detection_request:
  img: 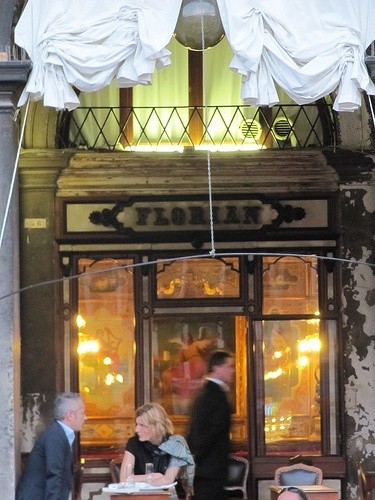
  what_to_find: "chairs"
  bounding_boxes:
[110,451,322,500]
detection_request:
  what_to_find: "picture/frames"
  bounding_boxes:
[151,318,240,418]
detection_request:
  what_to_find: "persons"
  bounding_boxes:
[277,487,307,500]
[119,403,195,500]
[187,351,235,500]
[17,392,86,500]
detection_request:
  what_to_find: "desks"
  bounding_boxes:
[268,485,338,500]
[105,481,178,500]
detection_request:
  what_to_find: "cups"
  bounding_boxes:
[126,464,134,488]
[145,463,154,484]
